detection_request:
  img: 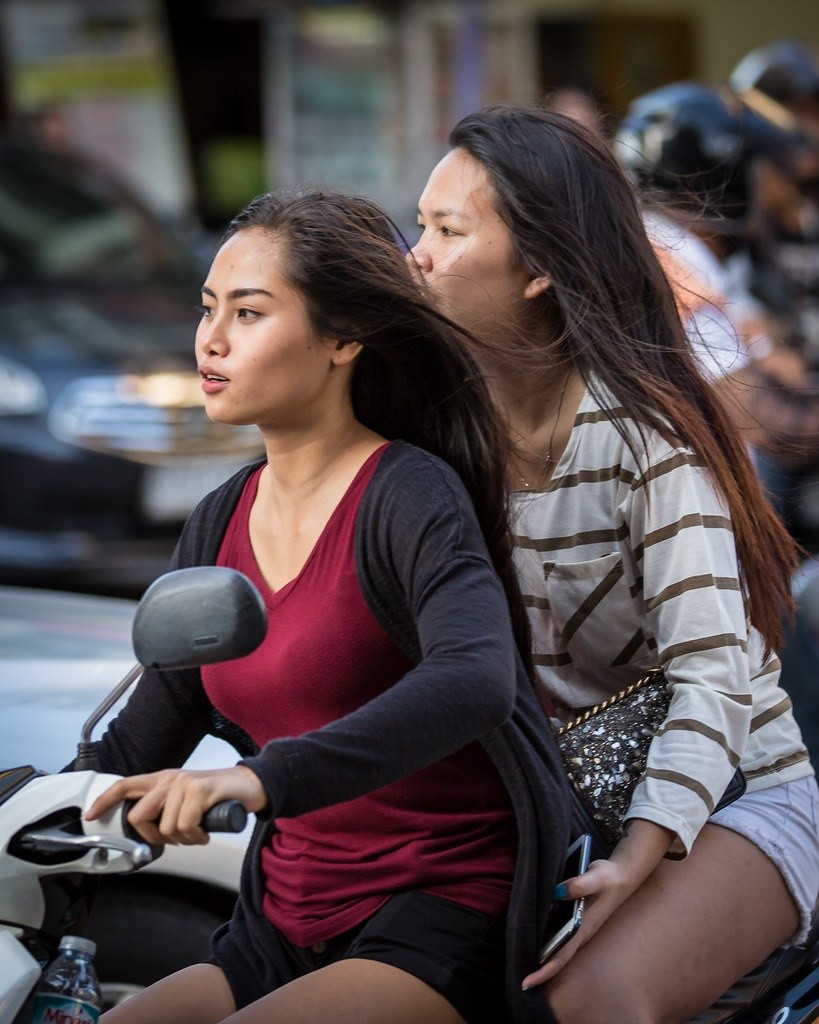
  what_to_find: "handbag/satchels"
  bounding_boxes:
[555,668,746,848]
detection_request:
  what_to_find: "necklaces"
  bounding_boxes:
[511,366,573,492]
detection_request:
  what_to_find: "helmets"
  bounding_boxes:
[729,40,818,141]
[616,86,760,226]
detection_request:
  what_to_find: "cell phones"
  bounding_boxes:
[539,834,593,966]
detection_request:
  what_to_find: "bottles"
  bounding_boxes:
[23,937,104,1024]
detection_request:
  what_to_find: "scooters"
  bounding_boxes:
[2,565,819,1024]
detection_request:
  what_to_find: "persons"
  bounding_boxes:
[404,105,819,1024]
[540,64,819,562]
[63,191,620,1024]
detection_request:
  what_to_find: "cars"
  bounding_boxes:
[0,125,268,594]
